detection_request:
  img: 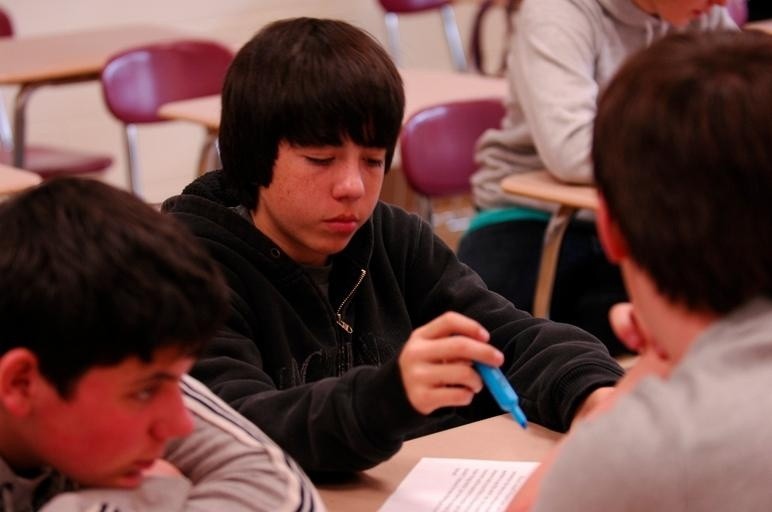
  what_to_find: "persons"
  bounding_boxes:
[504,27,772,512]
[0,177,327,512]
[459,0,743,360]
[160,18,625,487]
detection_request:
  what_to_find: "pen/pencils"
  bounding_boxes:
[450,332,528,430]
